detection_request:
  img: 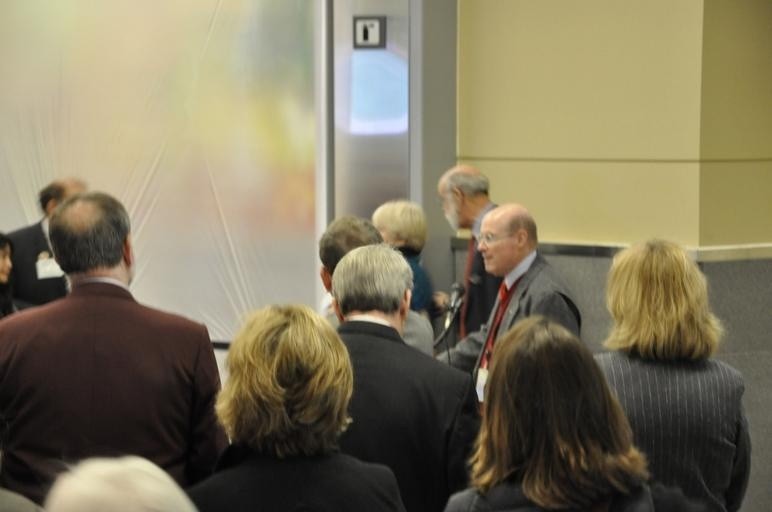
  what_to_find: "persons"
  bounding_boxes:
[0,191,230,507]
[370,199,434,328]
[318,215,434,356]
[437,165,505,350]
[5,177,88,305]
[435,204,582,415]
[445,313,656,512]
[42,454,199,512]
[331,243,480,511]
[186,301,406,512]
[593,238,752,512]
[0,232,14,317]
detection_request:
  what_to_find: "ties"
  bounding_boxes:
[457,235,475,341]
[480,275,524,369]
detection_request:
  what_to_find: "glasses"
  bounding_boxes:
[472,229,519,243]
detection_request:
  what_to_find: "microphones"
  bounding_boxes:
[446,282,465,311]
